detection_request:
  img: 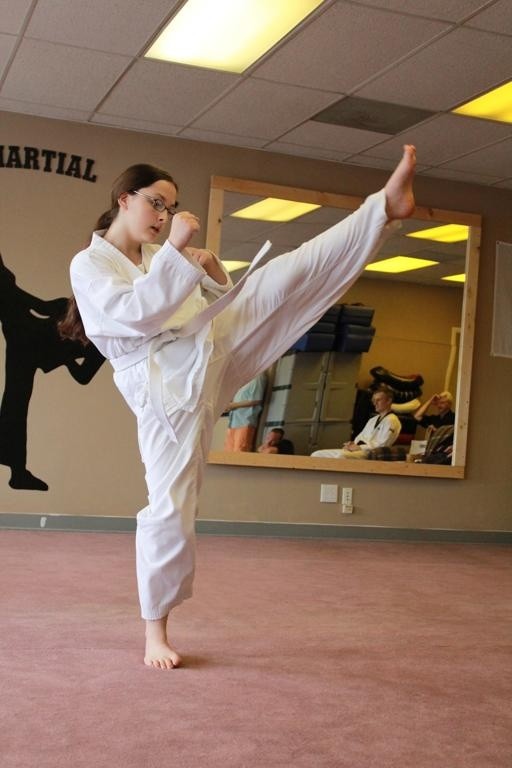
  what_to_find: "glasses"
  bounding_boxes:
[133,190,177,214]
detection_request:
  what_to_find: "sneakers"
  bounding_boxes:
[9,470,49,490]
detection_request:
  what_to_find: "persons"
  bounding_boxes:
[70,144,421,668]
[415,391,455,447]
[311,386,403,462]
[224,372,267,452]
[0,254,107,491]
[257,428,294,455]
[422,433,452,465]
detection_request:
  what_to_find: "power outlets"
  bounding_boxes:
[342,488,354,514]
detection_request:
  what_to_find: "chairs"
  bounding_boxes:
[367,433,416,461]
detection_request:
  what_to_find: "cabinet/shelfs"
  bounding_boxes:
[261,351,363,456]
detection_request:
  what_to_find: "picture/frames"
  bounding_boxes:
[489,240,512,359]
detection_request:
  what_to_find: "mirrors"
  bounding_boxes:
[202,173,484,481]
